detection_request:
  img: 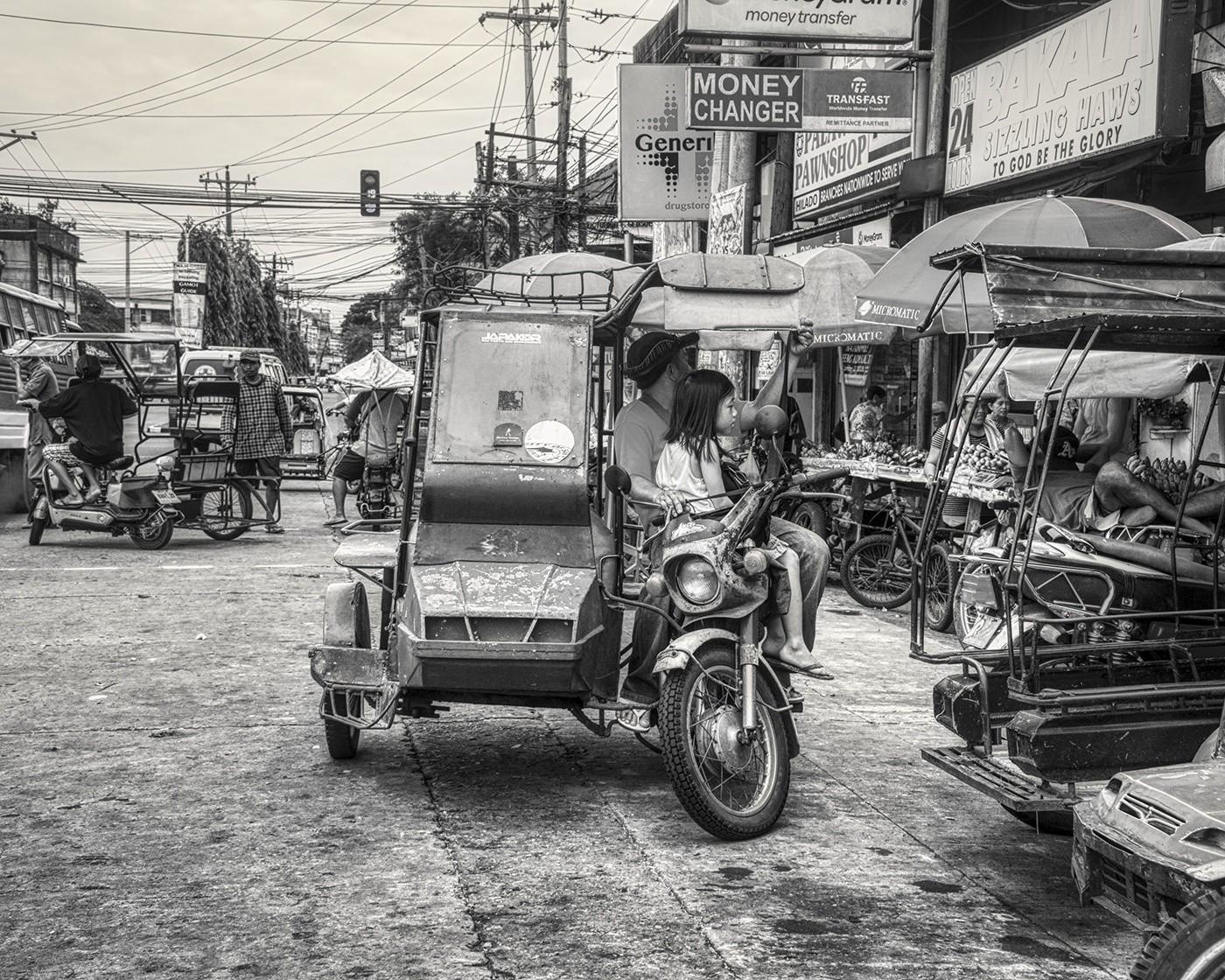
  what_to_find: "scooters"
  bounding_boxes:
[326,406,404,532]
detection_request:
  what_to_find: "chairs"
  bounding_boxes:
[1008,517,1225,662]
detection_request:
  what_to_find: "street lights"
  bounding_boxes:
[101,183,275,263]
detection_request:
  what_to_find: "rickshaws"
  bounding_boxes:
[913,242,1225,831]
[15,330,282,550]
[303,249,857,843]
[1067,707,1224,980]
[172,373,330,481]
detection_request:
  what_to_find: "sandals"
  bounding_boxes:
[1119,506,1156,527]
[1196,537,1225,561]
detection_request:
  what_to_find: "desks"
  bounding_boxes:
[798,455,943,540]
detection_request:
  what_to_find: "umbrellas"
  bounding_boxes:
[624,189,1225,401]
[454,250,650,321]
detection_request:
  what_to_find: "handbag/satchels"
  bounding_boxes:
[831,418,851,443]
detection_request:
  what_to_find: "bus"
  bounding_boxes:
[0,281,84,513]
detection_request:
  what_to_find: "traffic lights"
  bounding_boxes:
[360,169,381,216]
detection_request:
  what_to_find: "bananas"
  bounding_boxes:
[1126,455,1210,495]
[799,432,929,468]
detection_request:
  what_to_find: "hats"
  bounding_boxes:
[239,349,261,361]
[76,355,101,374]
[1,339,41,355]
[1037,425,1080,471]
[623,332,701,381]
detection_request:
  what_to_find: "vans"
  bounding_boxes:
[168,348,294,438]
[122,327,183,399]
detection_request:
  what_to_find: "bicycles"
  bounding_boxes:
[840,483,958,632]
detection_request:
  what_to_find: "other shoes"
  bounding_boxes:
[240,522,252,532]
[265,525,284,533]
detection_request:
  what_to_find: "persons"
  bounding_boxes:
[219,348,293,534]
[2,339,60,530]
[293,376,318,429]
[831,385,1133,561]
[323,386,408,525]
[613,332,834,733]
[23,353,140,508]
[1002,424,1224,567]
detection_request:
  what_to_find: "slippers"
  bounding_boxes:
[615,697,651,733]
[781,663,833,679]
[323,518,347,528]
[783,685,805,703]
[84,495,106,506]
[764,656,787,667]
[50,498,83,507]
